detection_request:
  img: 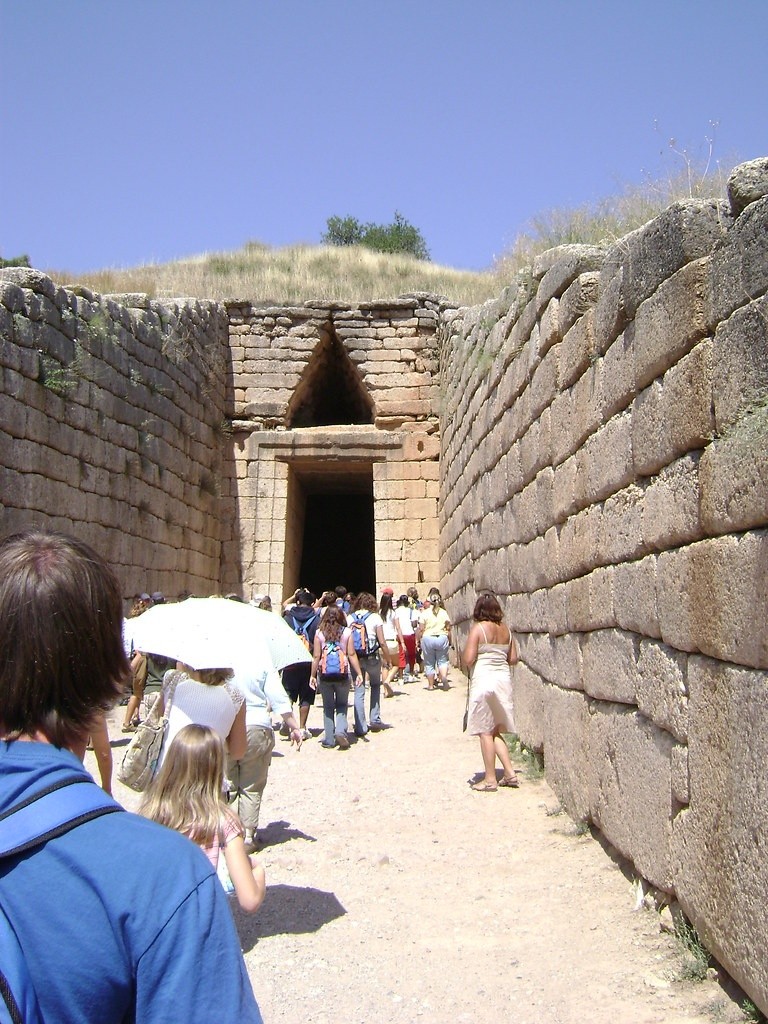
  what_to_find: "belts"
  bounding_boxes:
[386,638,398,641]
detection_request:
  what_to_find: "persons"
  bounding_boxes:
[135,725,266,913]
[151,660,304,873]
[462,592,519,791]
[278,585,456,751]
[0,535,259,1024]
[122,592,272,732]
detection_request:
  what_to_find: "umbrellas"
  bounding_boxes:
[126,597,315,678]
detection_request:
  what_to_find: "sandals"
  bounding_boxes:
[498,776,520,788]
[472,779,497,791]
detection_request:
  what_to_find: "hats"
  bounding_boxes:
[140,593,151,601]
[225,592,241,599]
[250,594,265,607]
[427,595,440,603]
[380,586,394,594]
[153,592,164,600]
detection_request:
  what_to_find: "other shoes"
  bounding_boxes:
[370,723,390,732]
[336,732,350,749]
[121,723,138,733]
[442,677,450,691]
[244,836,265,854]
[132,720,144,727]
[383,682,394,698]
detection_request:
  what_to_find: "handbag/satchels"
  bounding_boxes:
[463,711,468,732]
[117,669,185,793]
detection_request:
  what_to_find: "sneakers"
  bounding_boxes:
[409,675,420,682]
[279,720,290,736]
[398,679,404,686]
[299,726,312,740]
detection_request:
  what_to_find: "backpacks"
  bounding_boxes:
[284,615,317,673]
[350,611,380,656]
[320,626,348,680]
